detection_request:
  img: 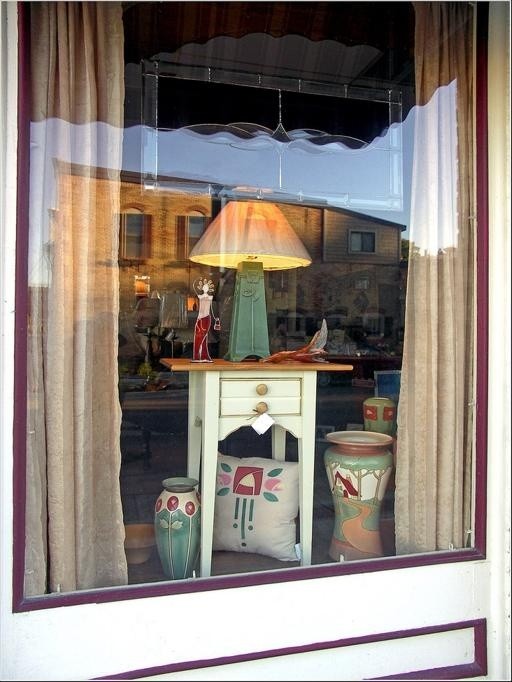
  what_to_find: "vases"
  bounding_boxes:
[321,430,393,562]
[363,397,396,433]
[152,477,200,578]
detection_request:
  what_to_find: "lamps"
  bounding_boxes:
[186,200,314,361]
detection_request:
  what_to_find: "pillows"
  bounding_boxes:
[212,448,300,564]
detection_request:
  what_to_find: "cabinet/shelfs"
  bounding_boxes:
[158,357,353,577]
